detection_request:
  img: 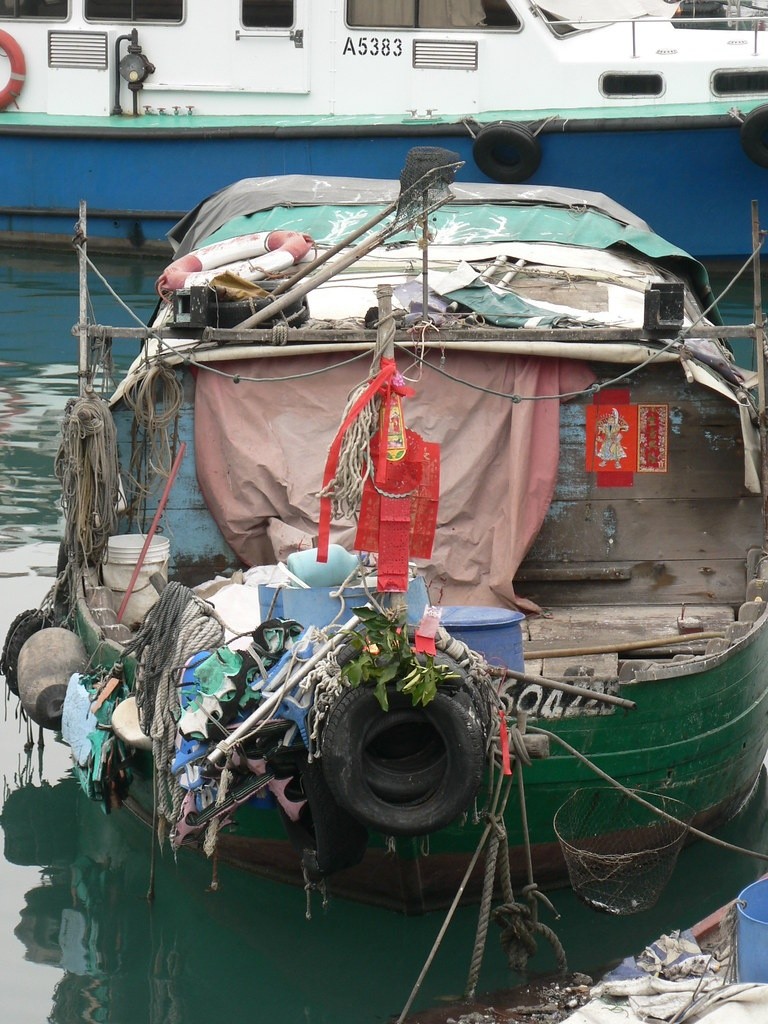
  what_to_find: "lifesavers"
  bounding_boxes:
[156,226,313,300]
[263,644,489,878]
[0,30,26,113]
[470,119,539,182]
[741,107,768,168]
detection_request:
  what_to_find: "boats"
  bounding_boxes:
[0,0,768,264]
[68,174,765,916]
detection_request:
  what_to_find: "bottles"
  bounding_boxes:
[286,543,371,587]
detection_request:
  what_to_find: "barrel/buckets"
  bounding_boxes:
[281,574,432,626]
[736,877,768,983]
[257,583,282,626]
[427,604,526,678]
[100,534,171,628]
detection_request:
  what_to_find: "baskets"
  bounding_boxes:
[553,787,697,917]
